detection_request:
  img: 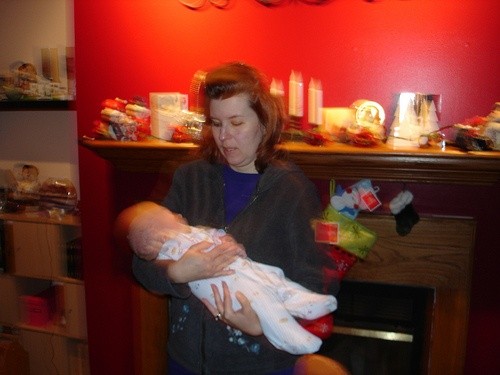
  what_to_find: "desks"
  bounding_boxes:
[77,137,500,375]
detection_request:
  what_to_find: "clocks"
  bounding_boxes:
[350,99,386,127]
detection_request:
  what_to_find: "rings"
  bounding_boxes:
[216,313,221,320]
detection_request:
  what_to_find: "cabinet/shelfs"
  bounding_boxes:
[0,99,86,343]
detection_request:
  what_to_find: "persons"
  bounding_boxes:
[107,62,322,375]
[113,198,337,354]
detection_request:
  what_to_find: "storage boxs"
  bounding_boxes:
[150,91,187,141]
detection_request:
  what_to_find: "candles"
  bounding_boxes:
[271,70,322,128]
